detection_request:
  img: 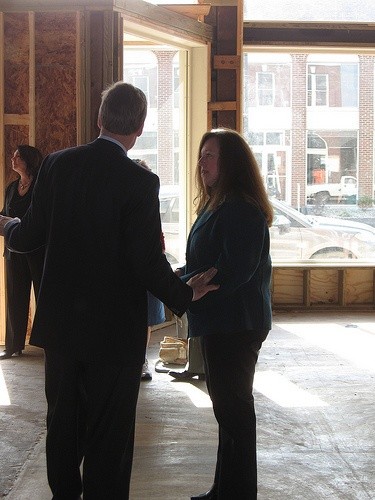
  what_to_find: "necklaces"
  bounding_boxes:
[19,181,29,190]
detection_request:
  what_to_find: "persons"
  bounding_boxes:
[0,143,43,359]
[0,81,219,500]
[130,127,273,500]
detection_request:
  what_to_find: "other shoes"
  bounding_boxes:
[140,370,152,380]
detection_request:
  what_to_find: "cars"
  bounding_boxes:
[158,184,375,268]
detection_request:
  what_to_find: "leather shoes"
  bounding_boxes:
[169,370,205,380]
[191,483,220,500]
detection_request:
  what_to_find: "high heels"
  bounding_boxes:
[0,347,22,359]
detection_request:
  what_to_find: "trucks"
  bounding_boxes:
[305,176,358,206]
